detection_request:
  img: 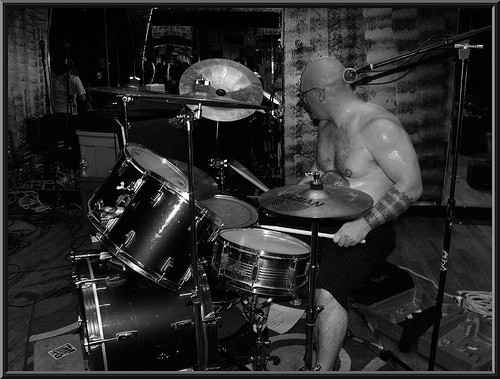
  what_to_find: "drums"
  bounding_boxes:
[194,192,258,228]
[208,227,313,301]
[79,141,191,234]
[70,250,221,372]
[98,170,225,294]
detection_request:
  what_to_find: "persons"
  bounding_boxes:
[299,58,423,371]
[50,58,86,115]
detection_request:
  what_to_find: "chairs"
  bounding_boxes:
[344,265,415,359]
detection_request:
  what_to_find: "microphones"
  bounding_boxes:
[342,67,357,83]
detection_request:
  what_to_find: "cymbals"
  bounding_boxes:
[82,84,157,97]
[167,158,219,201]
[138,94,272,110]
[258,182,375,220]
[177,58,266,123]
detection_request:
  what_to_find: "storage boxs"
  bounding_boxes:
[76,175,105,223]
[75,128,118,176]
[368,286,492,371]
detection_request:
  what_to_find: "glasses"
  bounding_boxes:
[298,88,325,100]
